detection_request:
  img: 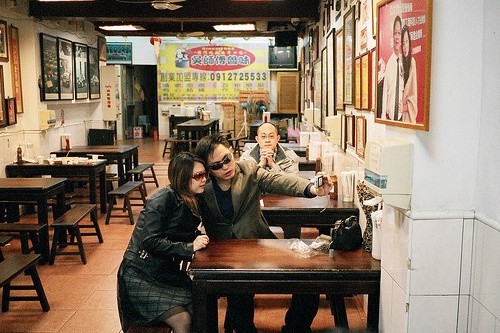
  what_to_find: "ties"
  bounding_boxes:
[394,58,399,120]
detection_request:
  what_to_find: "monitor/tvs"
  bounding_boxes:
[268,45,296,68]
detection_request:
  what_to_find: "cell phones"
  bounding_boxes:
[314,175,323,187]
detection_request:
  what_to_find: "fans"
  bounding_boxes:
[117,0,187,10]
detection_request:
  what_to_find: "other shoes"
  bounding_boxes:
[281,324,311,333]
[225,324,258,333]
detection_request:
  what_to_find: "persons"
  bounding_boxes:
[239,122,302,240]
[193,133,332,333]
[381,16,418,124]
[117,152,210,333]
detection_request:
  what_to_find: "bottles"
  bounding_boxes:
[17,147,23,164]
[264,114,267,122]
[306,145,309,160]
[197,106,204,120]
[65,136,70,150]
[329,175,337,200]
[315,157,321,176]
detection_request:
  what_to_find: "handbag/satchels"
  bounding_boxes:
[334,215,362,250]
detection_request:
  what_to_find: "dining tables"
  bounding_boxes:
[0,144,140,261]
[189,142,381,332]
[176,117,220,148]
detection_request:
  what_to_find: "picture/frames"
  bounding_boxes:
[0,20,100,127]
[299,0,433,157]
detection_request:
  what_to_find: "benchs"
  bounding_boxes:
[160,132,247,157]
[0,162,159,311]
[125,323,171,333]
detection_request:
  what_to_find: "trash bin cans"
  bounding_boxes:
[89,128,116,161]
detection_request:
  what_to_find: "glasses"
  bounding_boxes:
[191,170,207,182]
[208,151,232,171]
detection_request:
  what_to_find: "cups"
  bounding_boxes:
[51,154,56,159]
[92,155,98,161]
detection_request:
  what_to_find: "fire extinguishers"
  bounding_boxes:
[154,129,157,140]
